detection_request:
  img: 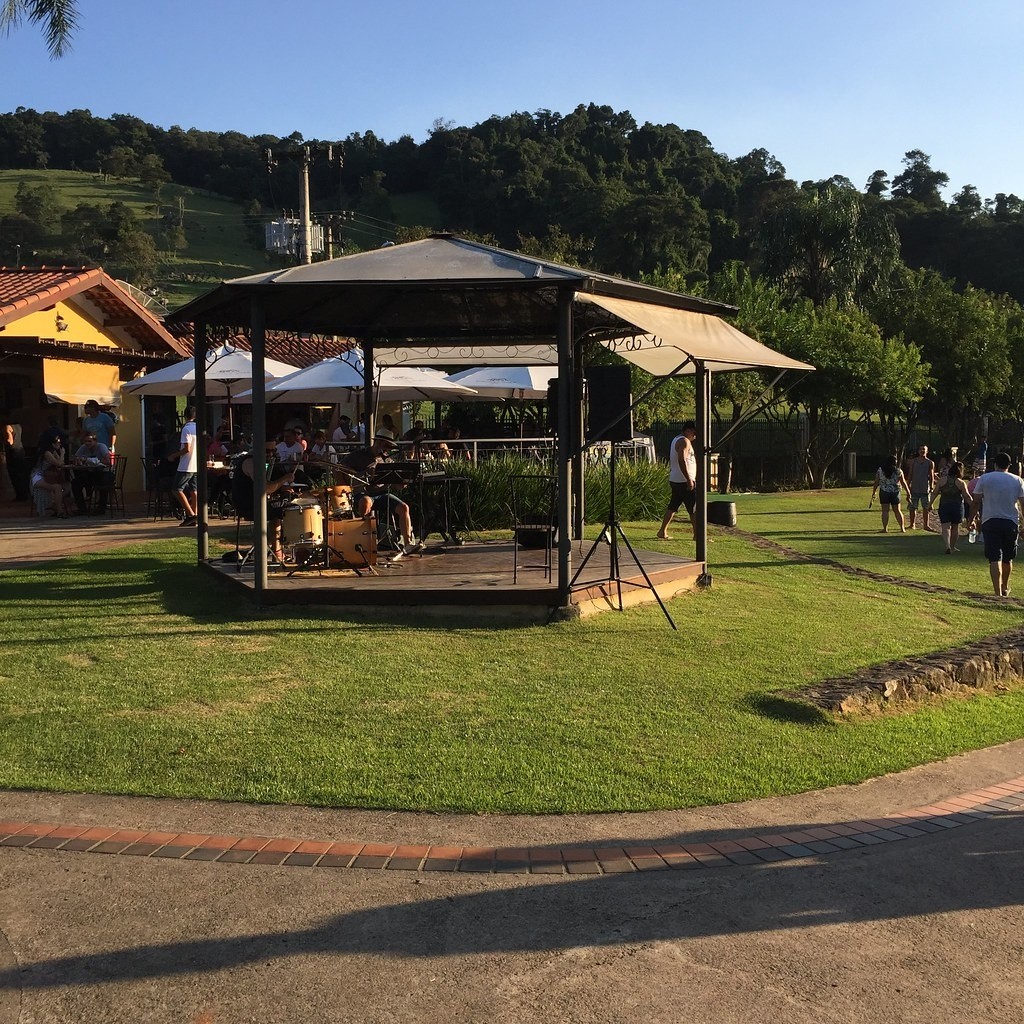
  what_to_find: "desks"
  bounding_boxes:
[408,478,470,547]
[58,465,110,516]
[207,468,228,478]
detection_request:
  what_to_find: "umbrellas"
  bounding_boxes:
[120,338,302,441]
[445,364,559,462]
[234,344,479,451]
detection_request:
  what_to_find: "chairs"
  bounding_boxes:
[88,453,127,518]
[509,475,559,584]
[140,457,173,523]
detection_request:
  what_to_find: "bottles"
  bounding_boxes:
[968,529,976,543]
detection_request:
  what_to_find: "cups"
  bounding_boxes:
[287,452,308,462]
[209,454,214,462]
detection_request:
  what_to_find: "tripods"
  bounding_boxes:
[369,434,440,558]
[238,460,362,576]
[546,443,677,632]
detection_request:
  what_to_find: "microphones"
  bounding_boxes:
[355,544,363,554]
[378,448,386,463]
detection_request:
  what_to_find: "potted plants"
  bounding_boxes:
[515,496,558,549]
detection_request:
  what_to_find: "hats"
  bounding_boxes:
[370,428,397,446]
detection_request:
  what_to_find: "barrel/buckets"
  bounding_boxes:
[707,501,737,526]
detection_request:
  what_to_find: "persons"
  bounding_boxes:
[656,421,700,539]
[937,440,1024,529]
[927,461,973,553]
[32,400,473,556]
[872,453,912,533]
[965,452,1024,597]
[905,445,936,531]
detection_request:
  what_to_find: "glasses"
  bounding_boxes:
[295,431,302,436]
[266,447,277,454]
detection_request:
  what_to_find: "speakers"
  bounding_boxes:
[587,364,633,443]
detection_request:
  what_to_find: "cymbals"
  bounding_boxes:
[277,458,304,464]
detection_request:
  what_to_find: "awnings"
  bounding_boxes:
[371,343,565,439]
[571,283,817,458]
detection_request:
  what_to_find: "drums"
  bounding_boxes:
[326,515,377,568]
[282,495,323,548]
[311,485,352,517]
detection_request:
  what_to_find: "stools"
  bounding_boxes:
[237,514,286,573]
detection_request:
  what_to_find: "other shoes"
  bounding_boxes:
[272,550,294,563]
[187,521,196,526]
[179,515,197,527]
[403,542,423,556]
[1002,588,1012,596]
